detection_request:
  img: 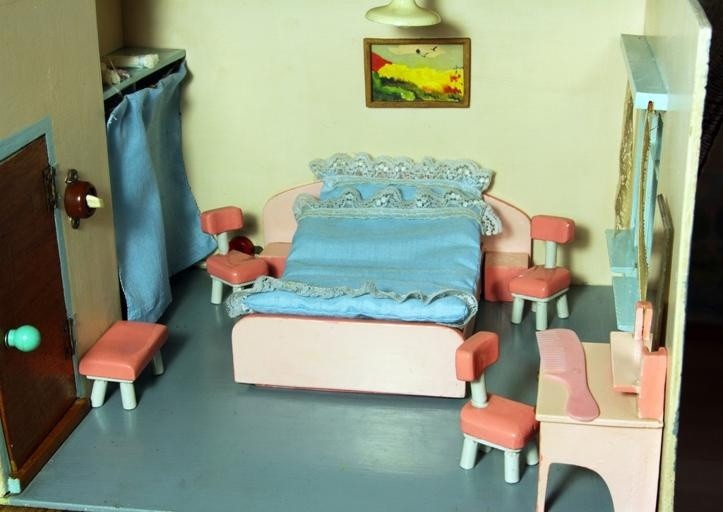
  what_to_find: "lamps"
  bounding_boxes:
[363,0,440,28]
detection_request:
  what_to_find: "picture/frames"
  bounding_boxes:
[363,37,471,107]
